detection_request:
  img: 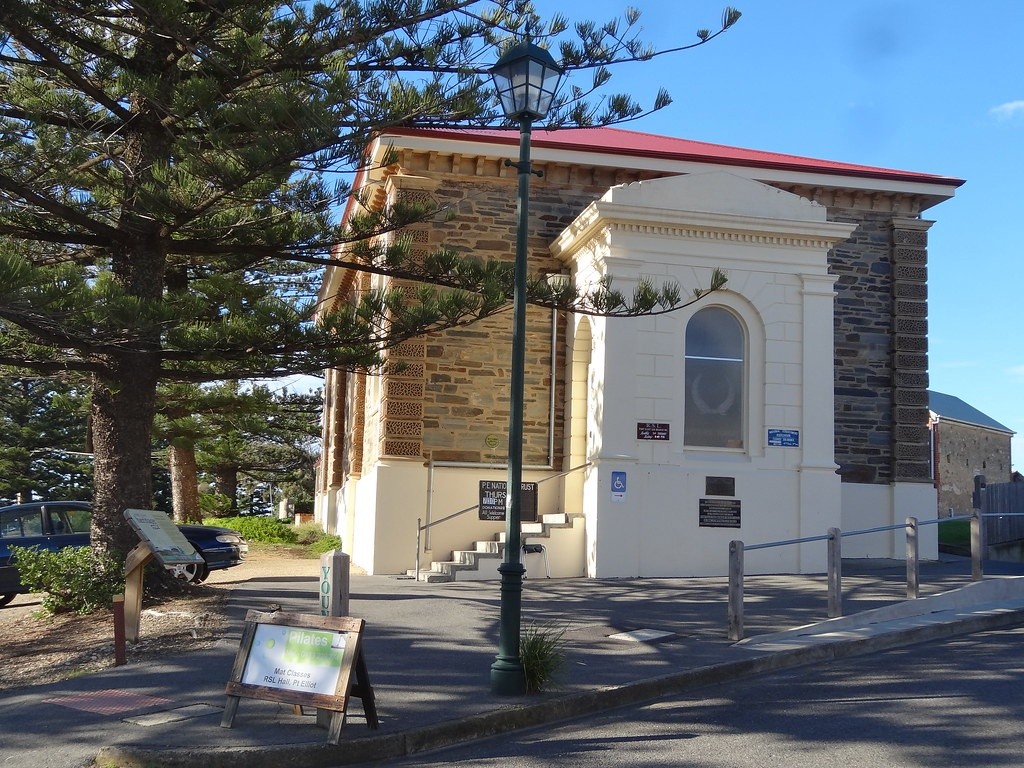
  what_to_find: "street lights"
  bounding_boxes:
[269,482,273,517]
[488,19,566,693]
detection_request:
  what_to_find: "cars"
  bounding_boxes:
[0,501,248,609]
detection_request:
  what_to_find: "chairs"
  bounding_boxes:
[56,521,71,533]
